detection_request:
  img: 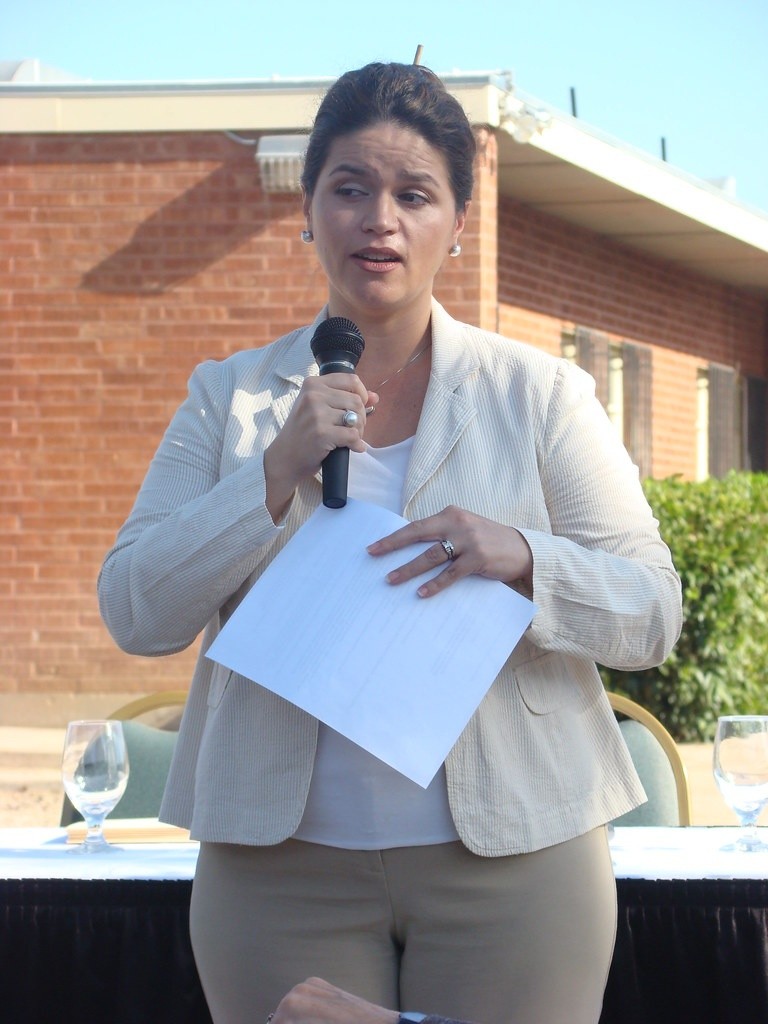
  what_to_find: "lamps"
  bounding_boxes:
[255,133,308,194]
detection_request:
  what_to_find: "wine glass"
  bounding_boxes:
[62,720,130,856]
[711,715,768,849]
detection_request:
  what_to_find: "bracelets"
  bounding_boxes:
[398,1012,474,1024]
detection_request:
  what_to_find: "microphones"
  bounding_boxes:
[309,316,365,511]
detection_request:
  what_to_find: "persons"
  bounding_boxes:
[97,62,683,1024]
[270,976,476,1024]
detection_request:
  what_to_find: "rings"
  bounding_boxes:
[440,539,455,560]
[342,408,358,428]
[265,1013,274,1024]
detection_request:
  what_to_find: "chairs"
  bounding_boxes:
[61,689,188,826]
[605,690,689,825]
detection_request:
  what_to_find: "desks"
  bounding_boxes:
[0,816,768,1024]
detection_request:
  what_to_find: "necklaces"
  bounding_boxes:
[365,343,432,415]
